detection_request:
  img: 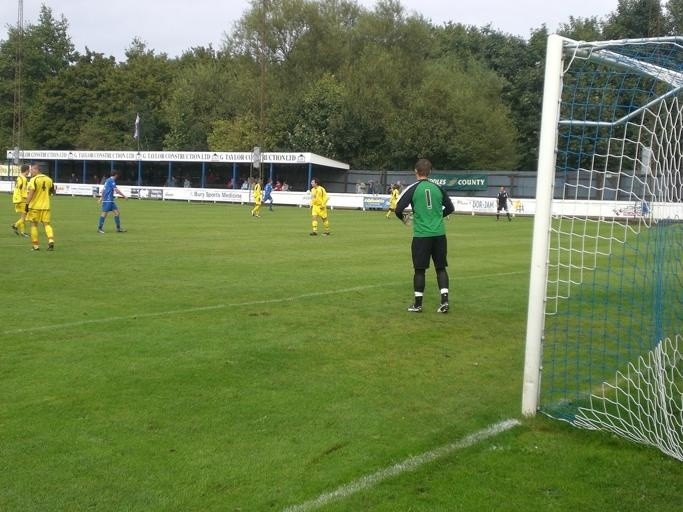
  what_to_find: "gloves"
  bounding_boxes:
[24,204,28,212]
[402,212,415,227]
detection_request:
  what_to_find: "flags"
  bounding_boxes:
[133,113,140,138]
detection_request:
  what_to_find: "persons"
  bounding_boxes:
[250,179,261,218]
[494,186,513,221]
[10,165,30,238]
[308,177,329,236]
[69,171,295,191]
[95,169,128,233]
[385,185,400,219]
[24,163,56,252]
[354,178,402,211]
[260,179,274,211]
[393,158,456,313]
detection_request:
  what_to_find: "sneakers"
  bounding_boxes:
[20,232,30,238]
[96,228,105,234]
[114,227,127,233]
[321,232,330,236]
[45,240,55,252]
[436,303,449,313]
[28,245,40,252]
[11,223,19,236]
[406,304,423,313]
[309,231,318,236]
